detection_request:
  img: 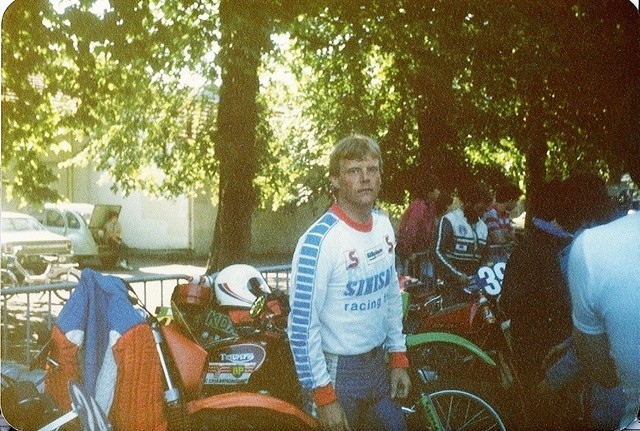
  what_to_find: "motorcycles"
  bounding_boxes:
[154,266,303,406]
[398,273,530,429]
[1,266,322,431]
[55,279,312,422]
[0,349,121,360]
[397,290,514,430]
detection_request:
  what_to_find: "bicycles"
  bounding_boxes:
[0,245,81,301]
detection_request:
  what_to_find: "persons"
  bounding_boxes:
[286,135,412,430]
[500,177,574,431]
[553,132,640,431]
[434,171,461,242]
[499,189,523,235]
[398,173,442,279]
[432,183,490,283]
[104,211,129,269]
[532,175,565,220]
[517,171,625,431]
[482,182,515,266]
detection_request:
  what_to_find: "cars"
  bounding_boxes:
[1,211,73,274]
[27,203,122,270]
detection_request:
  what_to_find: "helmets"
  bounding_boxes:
[214,262,271,308]
